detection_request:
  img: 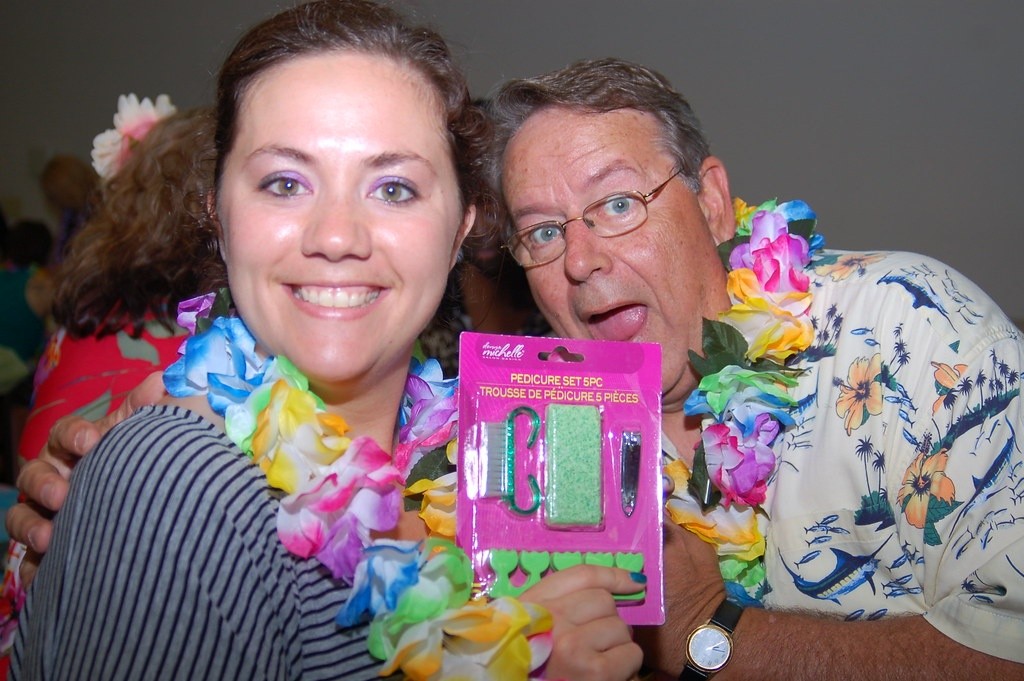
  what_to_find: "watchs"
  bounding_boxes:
[678,595,747,681]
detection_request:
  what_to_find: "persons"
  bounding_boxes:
[5,56,1024,681]
[0,0,645,681]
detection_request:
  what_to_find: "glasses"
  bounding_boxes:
[502,168,687,267]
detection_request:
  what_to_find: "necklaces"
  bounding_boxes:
[163,289,555,681]
[658,195,824,608]
